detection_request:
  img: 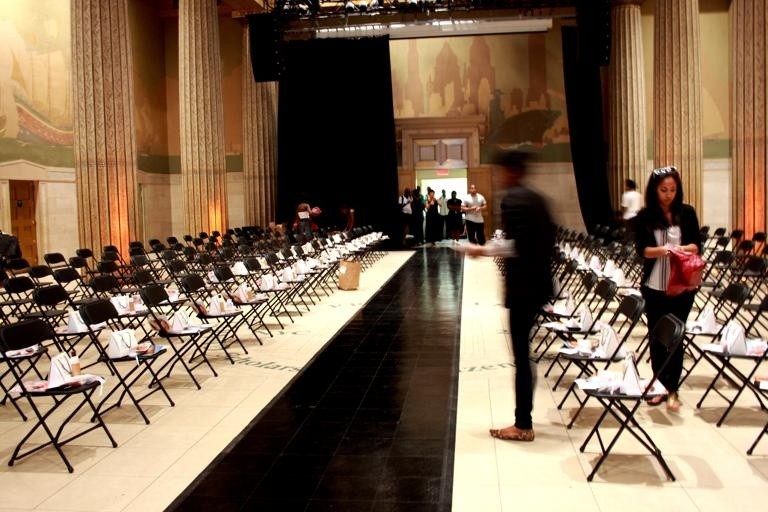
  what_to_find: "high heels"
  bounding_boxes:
[647,389,669,405]
[667,393,679,411]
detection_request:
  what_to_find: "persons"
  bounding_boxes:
[463,148,556,442]
[424,186,431,204]
[412,189,425,247]
[417,185,425,210]
[398,186,413,242]
[619,177,643,231]
[438,189,450,240]
[424,189,444,242]
[461,183,488,246]
[636,164,702,413]
[291,203,313,246]
[446,190,464,239]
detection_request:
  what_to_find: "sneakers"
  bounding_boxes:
[490,425,534,441]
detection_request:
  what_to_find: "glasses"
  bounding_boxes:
[653,166,676,180]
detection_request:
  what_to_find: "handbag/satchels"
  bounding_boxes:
[306,232,383,269]
[260,274,274,291]
[599,321,624,358]
[668,249,703,296]
[721,319,747,355]
[50,351,71,385]
[338,260,360,290]
[109,329,138,358]
[620,351,643,397]
[283,266,293,280]
[581,304,595,331]
[110,296,130,315]
[172,310,190,332]
[68,311,88,332]
[556,242,625,288]
[296,259,309,274]
[298,211,309,219]
[210,293,225,315]
[565,292,578,315]
[238,284,248,303]
